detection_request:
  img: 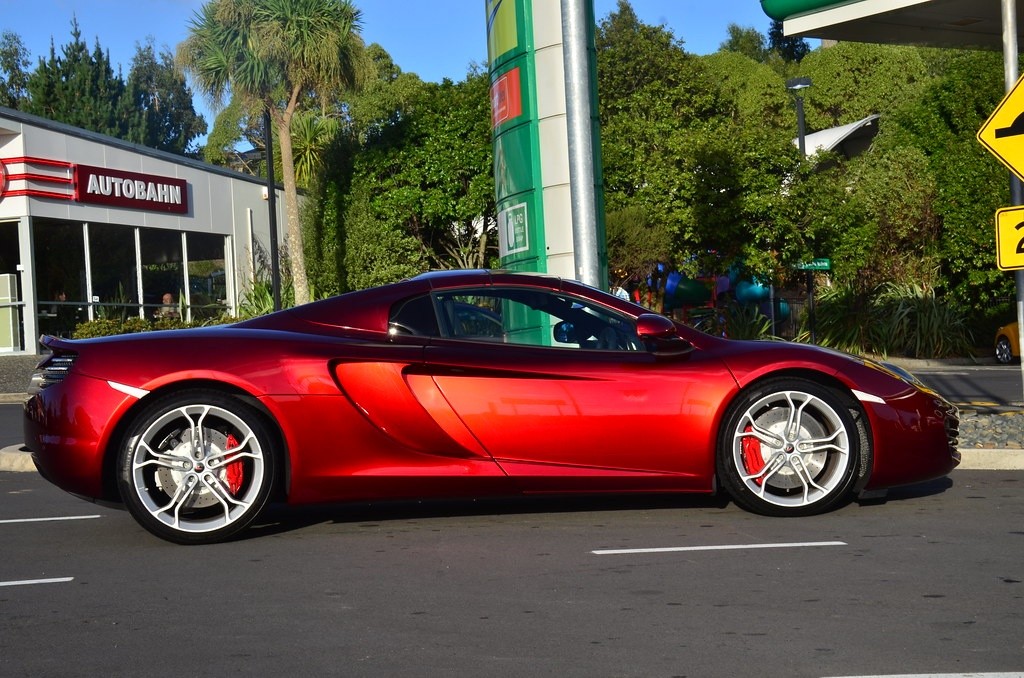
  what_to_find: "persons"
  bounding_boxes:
[51,290,67,337]
[161,293,180,314]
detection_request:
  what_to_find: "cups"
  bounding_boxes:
[42,310,47,318]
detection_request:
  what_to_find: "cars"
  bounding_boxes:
[993,320,1020,363]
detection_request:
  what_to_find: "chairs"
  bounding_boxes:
[441,296,466,337]
[45,300,76,339]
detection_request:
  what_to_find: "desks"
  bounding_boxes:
[37,313,79,338]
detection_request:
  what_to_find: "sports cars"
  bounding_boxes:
[19,270,963,546]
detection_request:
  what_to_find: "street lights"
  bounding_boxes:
[786,75,817,345]
